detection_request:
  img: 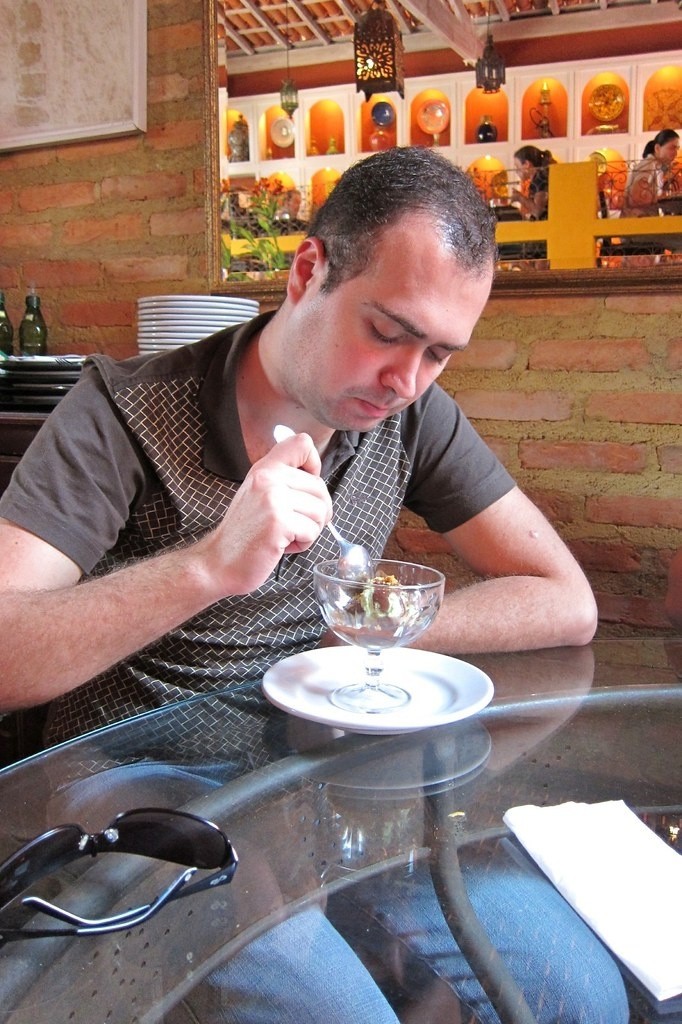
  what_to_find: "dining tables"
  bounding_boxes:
[0,635,682,1024]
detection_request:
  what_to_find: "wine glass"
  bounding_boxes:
[311,558,445,715]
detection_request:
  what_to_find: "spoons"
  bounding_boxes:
[273,423,374,589]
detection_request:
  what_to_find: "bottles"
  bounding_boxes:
[0,291,13,359]
[17,280,50,357]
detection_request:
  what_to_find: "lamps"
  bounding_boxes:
[353,0,404,98]
[280,0,298,118]
[475,0,506,95]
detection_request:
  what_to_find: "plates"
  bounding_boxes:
[418,99,448,134]
[589,82,626,121]
[370,102,394,127]
[261,645,496,735]
[270,118,294,148]
[136,295,261,365]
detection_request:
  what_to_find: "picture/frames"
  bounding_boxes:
[0,0,149,152]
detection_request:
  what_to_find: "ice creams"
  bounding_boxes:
[342,574,420,619]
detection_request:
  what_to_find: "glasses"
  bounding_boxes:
[0,807,239,938]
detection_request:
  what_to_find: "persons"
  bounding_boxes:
[511,145,557,260]
[0,147,632,1023]
[620,128,679,249]
